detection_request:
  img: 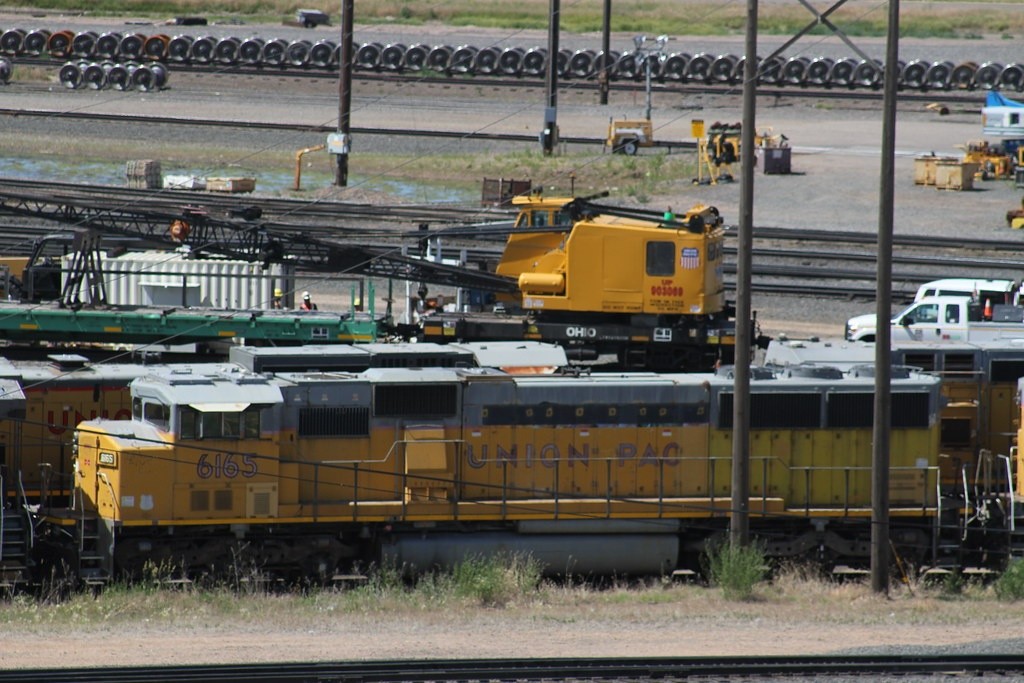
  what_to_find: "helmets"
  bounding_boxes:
[275,289,283,297]
[354,298,360,306]
[302,291,313,300]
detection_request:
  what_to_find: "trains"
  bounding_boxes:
[1,341,1024,580]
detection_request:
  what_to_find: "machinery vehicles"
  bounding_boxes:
[1,175,774,375]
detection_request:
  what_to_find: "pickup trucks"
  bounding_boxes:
[845,279,1024,364]
